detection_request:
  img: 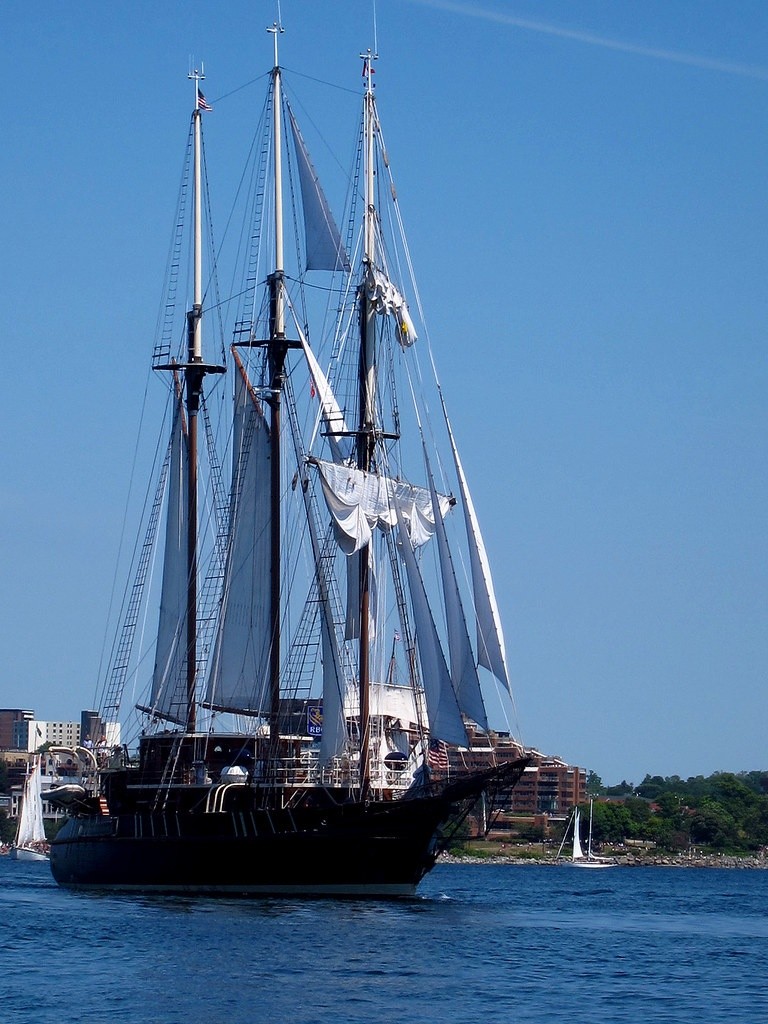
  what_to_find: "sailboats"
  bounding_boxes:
[11,753,48,861]
[38,0,534,899]
[562,806,619,869]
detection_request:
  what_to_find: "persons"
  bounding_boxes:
[429,739,448,772]
[83,734,108,764]
